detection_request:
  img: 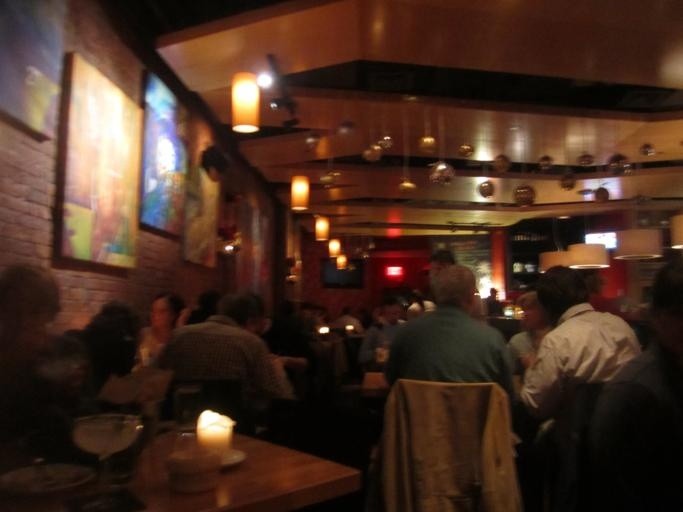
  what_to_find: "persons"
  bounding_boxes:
[0,249,683,511]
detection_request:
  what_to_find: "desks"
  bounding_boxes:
[0,425,361,512]
[484,314,528,342]
[359,372,522,403]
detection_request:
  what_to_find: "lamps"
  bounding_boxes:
[336,255,348,270]
[314,215,331,241]
[328,238,342,258]
[230,37,262,133]
[291,160,311,211]
